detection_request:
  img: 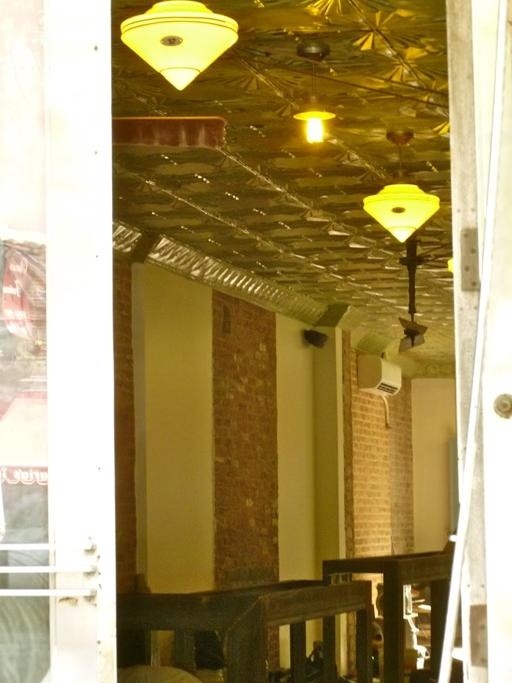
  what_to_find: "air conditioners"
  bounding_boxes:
[356,355,404,397]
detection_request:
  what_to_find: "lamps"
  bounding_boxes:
[293,45,337,144]
[121,2,240,91]
[362,130,439,239]
